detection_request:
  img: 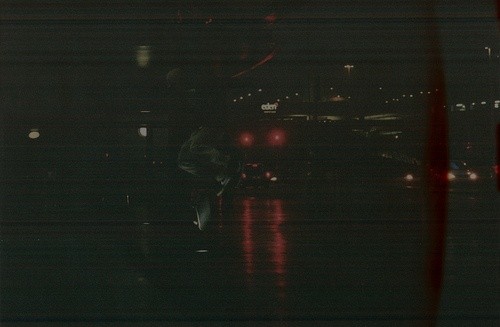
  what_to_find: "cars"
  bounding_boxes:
[399,163,497,188]
[239,164,273,188]
